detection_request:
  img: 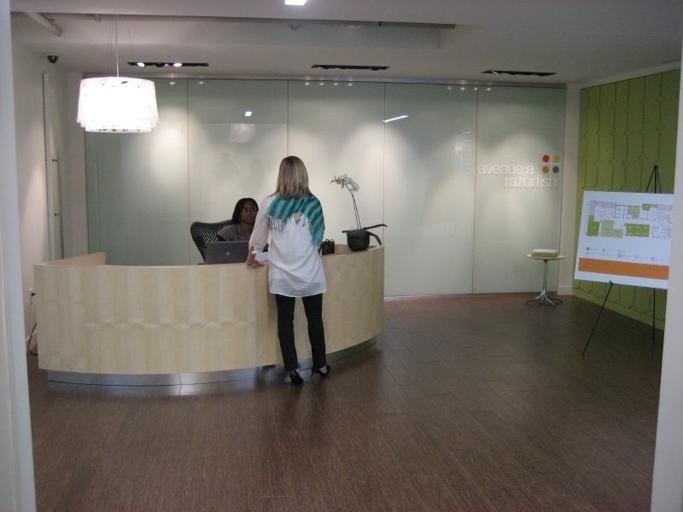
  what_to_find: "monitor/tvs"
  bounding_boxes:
[204,241,249,264]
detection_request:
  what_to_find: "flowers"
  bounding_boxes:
[329,173,388,245]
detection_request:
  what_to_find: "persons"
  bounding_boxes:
[246,155,331,387]
[215,195,259,242]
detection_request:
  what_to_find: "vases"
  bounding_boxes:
[347,231,370,251]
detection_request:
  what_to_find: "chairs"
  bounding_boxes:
[191,220,237,264]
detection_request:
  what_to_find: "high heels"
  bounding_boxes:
[310,365,331,378]
[289,371,303,385]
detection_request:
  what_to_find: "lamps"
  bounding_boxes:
[76,20,159,133]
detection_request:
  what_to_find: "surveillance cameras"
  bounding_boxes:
[47,55,59,65]
